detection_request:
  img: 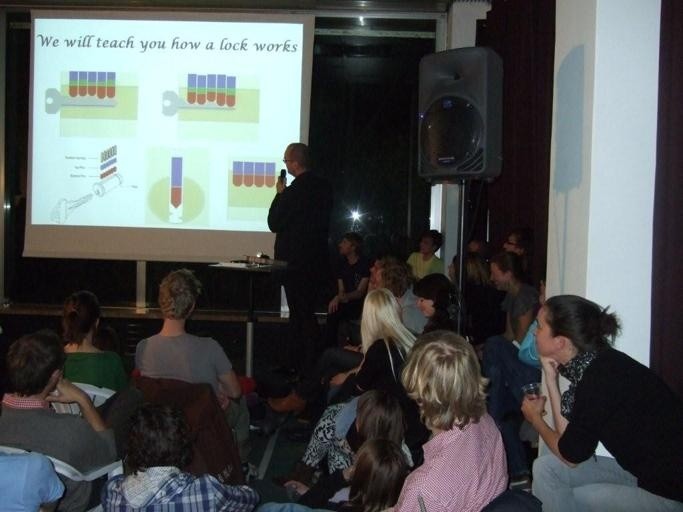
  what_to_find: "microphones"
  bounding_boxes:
[280,169,286,178]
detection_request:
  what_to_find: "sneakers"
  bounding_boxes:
[508,477,532,493]
[288,425,313,445]
[217,461,259,486]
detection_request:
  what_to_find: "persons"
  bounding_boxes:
[99,394,262,511]
[57,288,129,417]
[260,226,546,512]
[133,268,254,474]
[522,294,682,511]
[266,141,322,323]
[0,449,65,511]
[0,327,121,511]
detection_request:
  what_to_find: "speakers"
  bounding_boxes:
[417,46,503,186]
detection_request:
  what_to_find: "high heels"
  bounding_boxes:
[266,392,307,417]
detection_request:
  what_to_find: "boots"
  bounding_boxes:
[272,462,315,490]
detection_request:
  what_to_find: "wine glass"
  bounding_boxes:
[520,382,546,417]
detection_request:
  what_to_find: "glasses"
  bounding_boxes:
[505,238,521,248]
